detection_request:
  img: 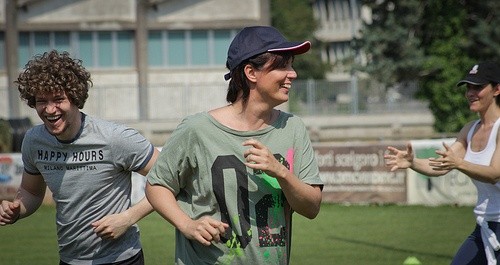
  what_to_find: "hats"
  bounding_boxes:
[456,60,500,87]
[224,25,312,80]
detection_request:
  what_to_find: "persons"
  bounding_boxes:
[0,48,162,265]
[382,64,500,265]
[145,25,325,265]
